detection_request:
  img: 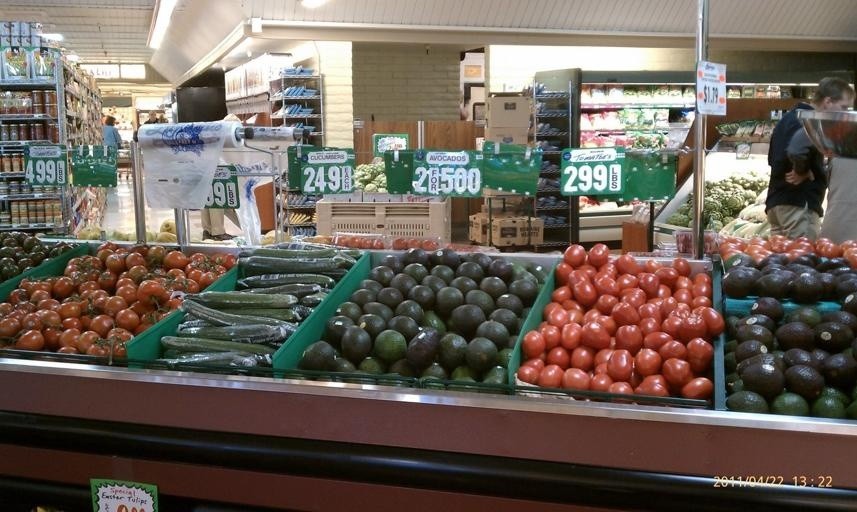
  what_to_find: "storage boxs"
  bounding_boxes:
[485,95,531,126]
[469,213,489,244]
[484,128,529,146]
[483,198,528,215]
[487,217,544,248]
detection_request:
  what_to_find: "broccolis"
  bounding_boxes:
[664,171,769,232]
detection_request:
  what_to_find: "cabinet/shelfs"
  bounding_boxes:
[2,236,857,508]
[2,43,73,240]
[579,83,696,250]
[528,79,572,252]
[268,73,325,147]
[274,152,321,241]
[62,59,108,237]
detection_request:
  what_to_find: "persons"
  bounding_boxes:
[818,154,857,239]
[765,77,857,239]
[103,116,122,146]
[136,110,166,125]
[202,209,234,241]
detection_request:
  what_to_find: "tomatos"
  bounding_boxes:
[0,240,235,356]
[522,244,727,402]
[722,236,853,268]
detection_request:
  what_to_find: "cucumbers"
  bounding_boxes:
[144,240,364,371]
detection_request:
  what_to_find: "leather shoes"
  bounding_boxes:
[209,232,236,243]
[202,229,212,240]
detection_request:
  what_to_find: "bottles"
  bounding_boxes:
[0,123,59,143]
[0,152,25,172]
[0,20,43,48]
[33,91,43,104]
[0,91,33,114]
[45,104,57,115]
[45,90,57,104]
[33,104,44,114]
[0,181,62,195]
[0,199,63,224]
